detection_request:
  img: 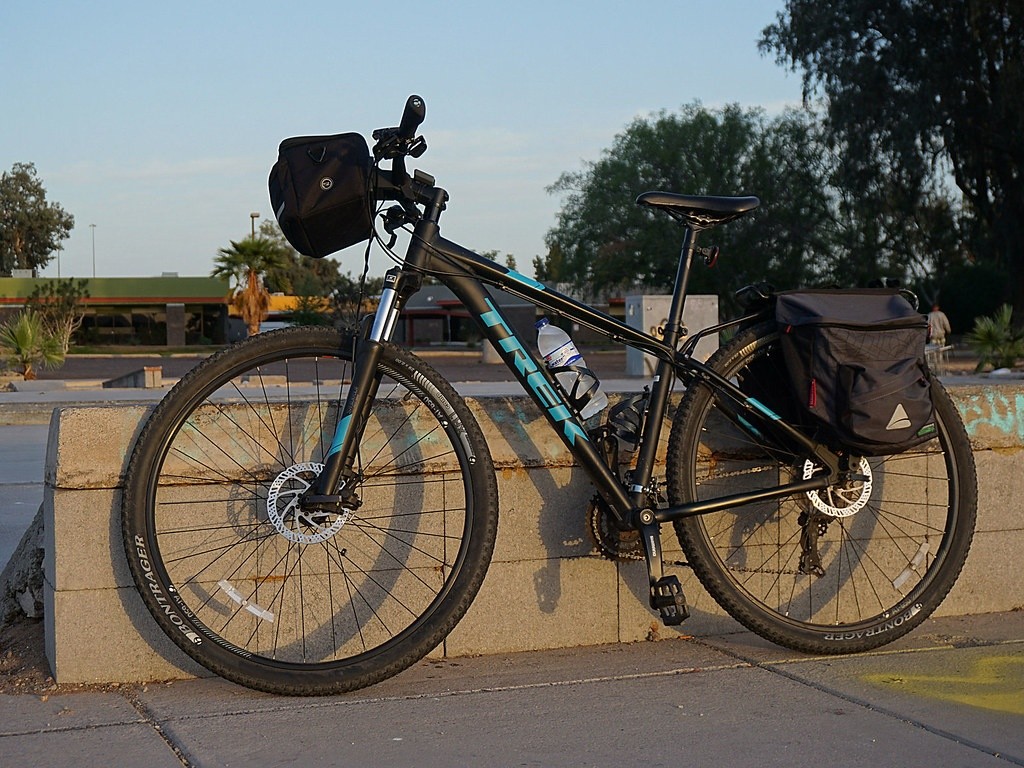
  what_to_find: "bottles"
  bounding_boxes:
[535,316,610,420]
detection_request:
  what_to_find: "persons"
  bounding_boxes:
[926,305,951,362]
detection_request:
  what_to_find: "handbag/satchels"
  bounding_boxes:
[267,132,375,259]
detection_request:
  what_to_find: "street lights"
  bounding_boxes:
[251,212,259,240]
[89,224,96,278]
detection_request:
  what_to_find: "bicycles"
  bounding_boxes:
[120,94,980,701]
